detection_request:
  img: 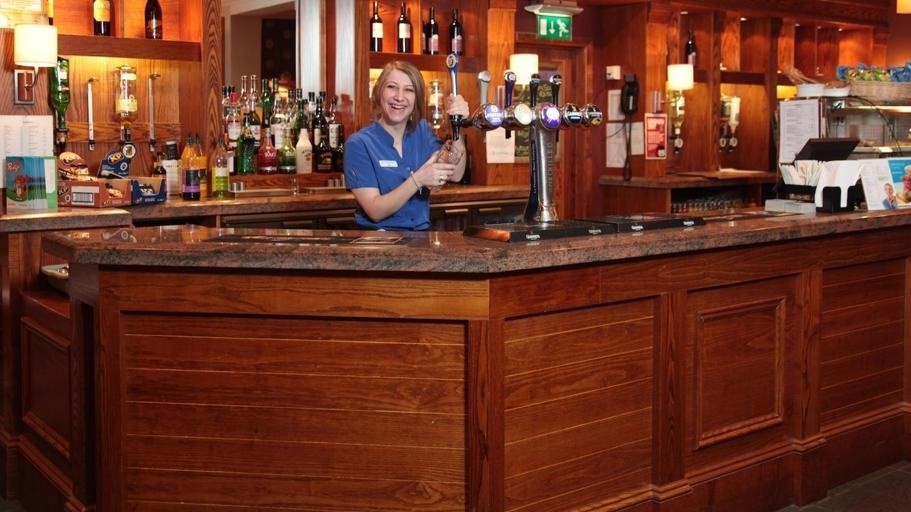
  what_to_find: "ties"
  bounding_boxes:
[792,137,860,165]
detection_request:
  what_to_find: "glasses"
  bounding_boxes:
[497,54,538,109]
[652,64,694,120]
[13,24,58,104]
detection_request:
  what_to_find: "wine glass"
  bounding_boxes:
[439,175,447,183]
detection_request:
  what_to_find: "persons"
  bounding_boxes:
[882,165,911,210]
[343,60,467,232]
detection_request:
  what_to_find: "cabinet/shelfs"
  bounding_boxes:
[685,30,697,68]
[720,96,741,138]
[425,80,445,135]
[144,0,163,39]
[671,94,686,137]
[114,65,137,140]
[151,75,345,201]
[93,0,111,36]
[370,0,464,56]
[51,58,70,128]
[672,195,733,214]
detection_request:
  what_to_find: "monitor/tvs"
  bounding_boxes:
[6,156,58,210]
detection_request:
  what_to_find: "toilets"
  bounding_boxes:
[620,74,639,115]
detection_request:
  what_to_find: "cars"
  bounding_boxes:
[424,139,465,193]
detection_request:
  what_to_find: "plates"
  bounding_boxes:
[366,0,487,182]
[44,1,205,188]
[667,3,875,177]
[821,97,911,153]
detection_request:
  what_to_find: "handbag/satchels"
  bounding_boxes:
[411,171,423,195]
